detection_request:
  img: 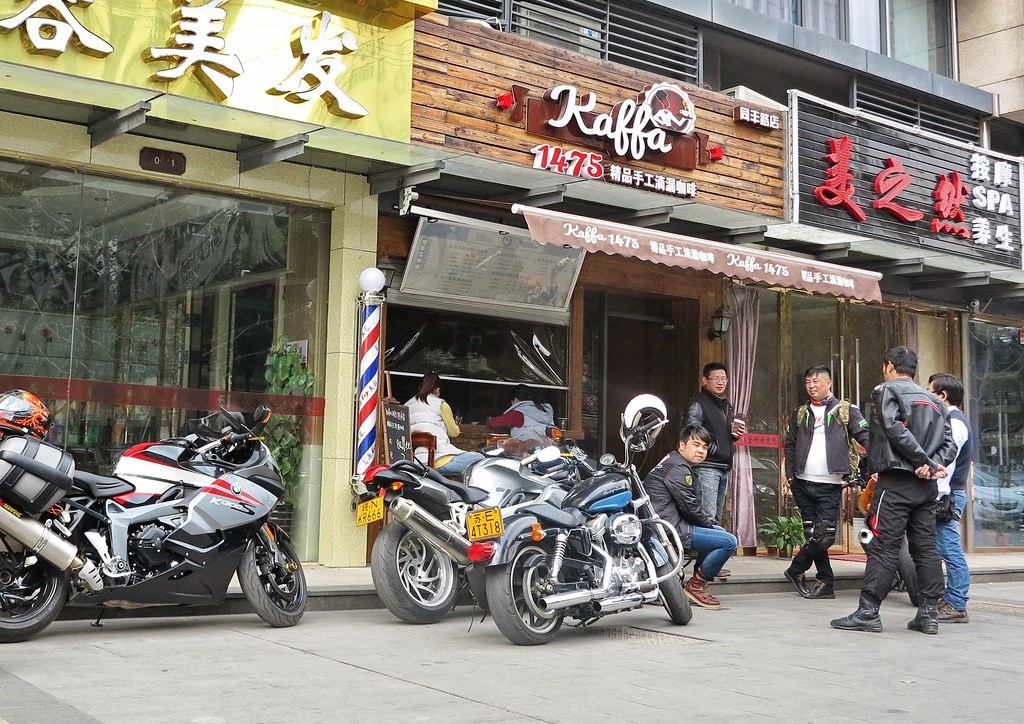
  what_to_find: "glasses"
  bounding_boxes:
[707,377,729,383]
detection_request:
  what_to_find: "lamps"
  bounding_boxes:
[708,302,734,342]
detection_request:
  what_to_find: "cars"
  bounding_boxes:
[970,459,1024,532]
[732,453,781,522]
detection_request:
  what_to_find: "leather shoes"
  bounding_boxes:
[806,583,835,599]
[785,567,811,595]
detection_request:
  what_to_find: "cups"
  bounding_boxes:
[556,417,568,431]
[730,418,745,436]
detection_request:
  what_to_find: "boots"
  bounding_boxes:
[830,595,882,631]
[907,596,939,634]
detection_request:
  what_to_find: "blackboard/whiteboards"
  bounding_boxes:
[380,401,414,465]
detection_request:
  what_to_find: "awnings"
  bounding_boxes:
[413,181,885,307]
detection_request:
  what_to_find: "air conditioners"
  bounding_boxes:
[720,85,787,111]
[511,3,601,58]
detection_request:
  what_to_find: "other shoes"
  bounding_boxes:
[715,569,732,578]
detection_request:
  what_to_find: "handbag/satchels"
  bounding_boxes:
[935,489,955,523]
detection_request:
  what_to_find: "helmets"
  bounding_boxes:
[0,389,56,443]
[619,393,667,452]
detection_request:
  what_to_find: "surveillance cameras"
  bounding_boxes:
[970,299,980,307]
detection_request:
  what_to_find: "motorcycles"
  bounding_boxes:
[0,388,309,646]
[855,478,921,607]
[362,435,602,625]
[466,392,696,647]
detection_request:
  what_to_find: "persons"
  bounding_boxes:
[927,371,974,624]
[400,369,486,474]
[486,384,555,453]
[785,365,871,602]
[682,362,746,579]
[644,424,737,610]
[828,346,957,635]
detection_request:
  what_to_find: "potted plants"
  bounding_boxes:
[756,518,780,556]
[758,515,805,560]
[981,518,1014,547]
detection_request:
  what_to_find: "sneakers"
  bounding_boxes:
[935,598,969,623]
[684,577,721,609]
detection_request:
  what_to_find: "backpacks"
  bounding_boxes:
[797,399,861,480]
